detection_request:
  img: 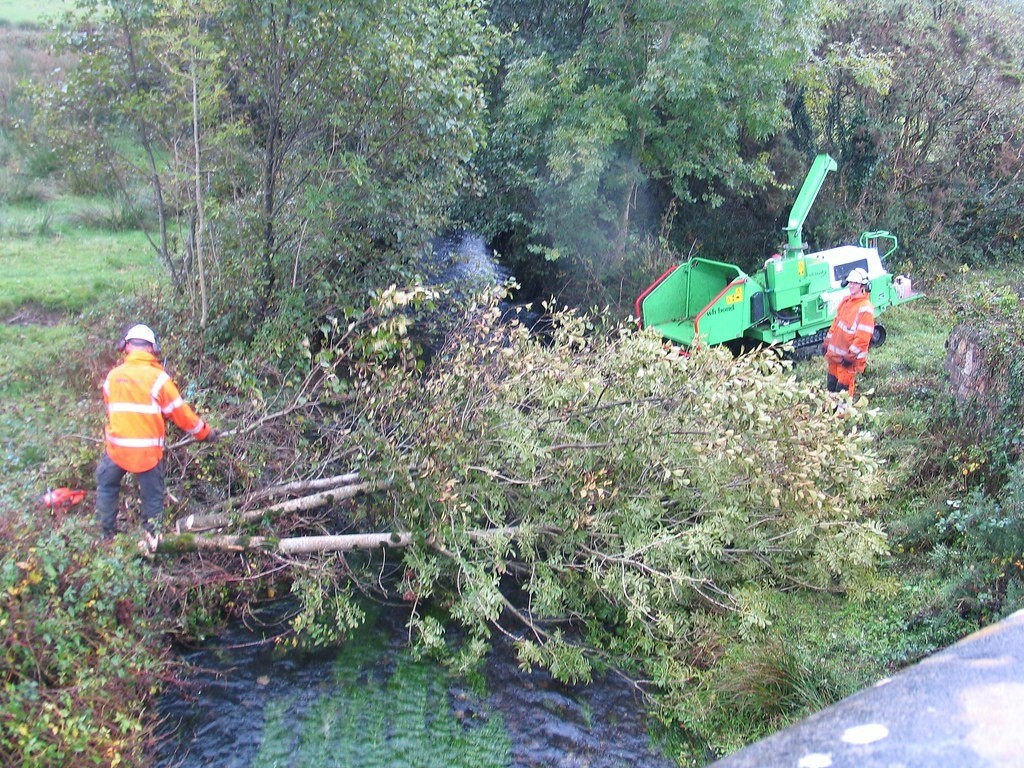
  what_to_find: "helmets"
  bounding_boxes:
[125,324,155,344]
[846,268,869,284]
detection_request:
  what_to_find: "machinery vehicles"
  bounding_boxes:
[635,152,927,369]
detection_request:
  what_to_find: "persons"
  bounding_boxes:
[821,267,876,405]
[96,324,219,563]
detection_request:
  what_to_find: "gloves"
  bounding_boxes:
[821,346,827,357]
[841,358,851,366]
[204,429,222,444]
